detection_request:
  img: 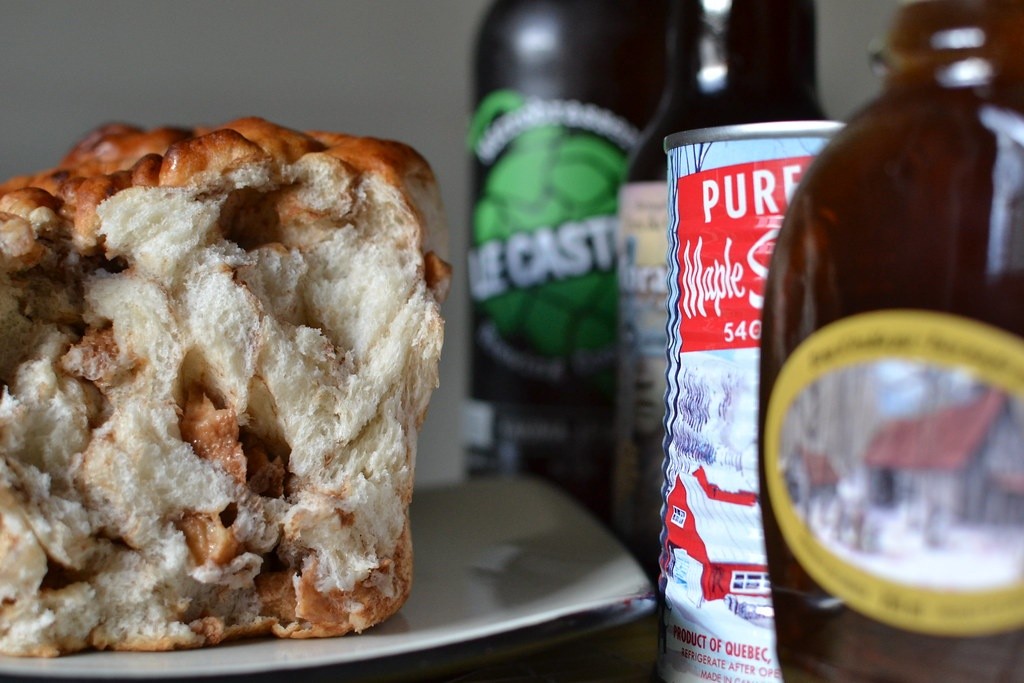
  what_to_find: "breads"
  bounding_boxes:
[0,113,452,662]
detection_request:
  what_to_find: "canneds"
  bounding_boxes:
[656,118,861,682]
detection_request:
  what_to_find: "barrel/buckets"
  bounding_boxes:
[652,121,847,683]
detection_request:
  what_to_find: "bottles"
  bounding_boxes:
[757,0,1023,683]
[611,0,828,581]
[463,0,671,527]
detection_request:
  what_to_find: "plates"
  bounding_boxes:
[0,475,657,682]
